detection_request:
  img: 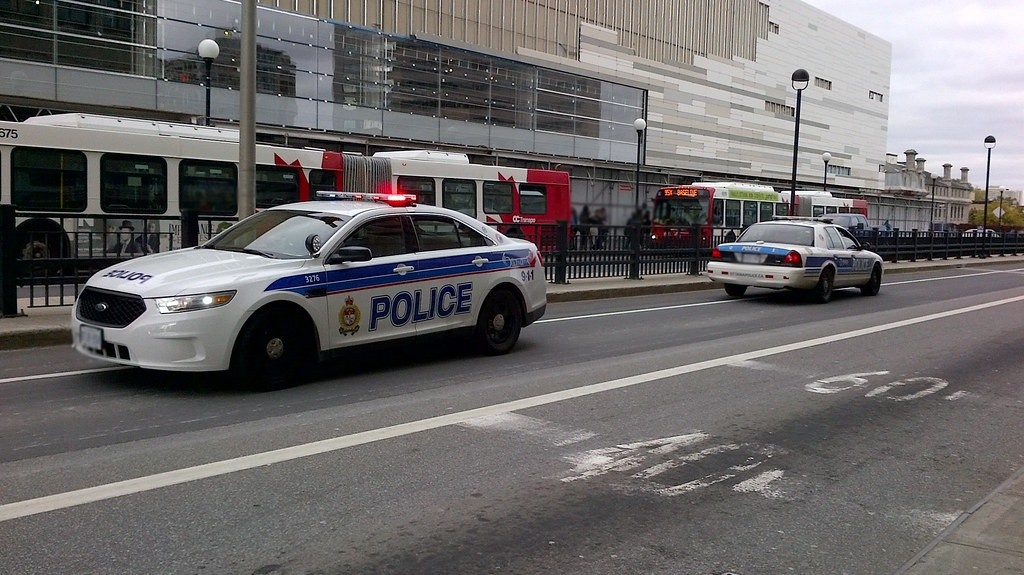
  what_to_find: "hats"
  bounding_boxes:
[141,168,166,188]
[216,222,233,233]
[118,220,135,231]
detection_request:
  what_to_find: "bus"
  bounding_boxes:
[649,183,799,250]
[0,110,571,278]
[779,190,870,220]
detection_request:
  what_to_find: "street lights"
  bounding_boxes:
[789,69,811,216]
[820,152,832,190]
[927,171,939,261]
[998,185,1005,229]
[628,119,646,281]
[198,37,220,128]
[978,135,997,259]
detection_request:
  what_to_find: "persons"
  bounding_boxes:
[884,220,891,237]
[572,203,652,250]
[106,221,160,253]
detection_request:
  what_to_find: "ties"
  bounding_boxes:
[120,243,126,253]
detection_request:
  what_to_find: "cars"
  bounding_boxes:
[962,228,1000,238]
[69,189,550,390]
[707,220,884,304]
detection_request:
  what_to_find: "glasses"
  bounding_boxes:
[108,225,115,230]
[144,176,163,185]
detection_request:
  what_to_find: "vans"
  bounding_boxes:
[818,212,869,233]
